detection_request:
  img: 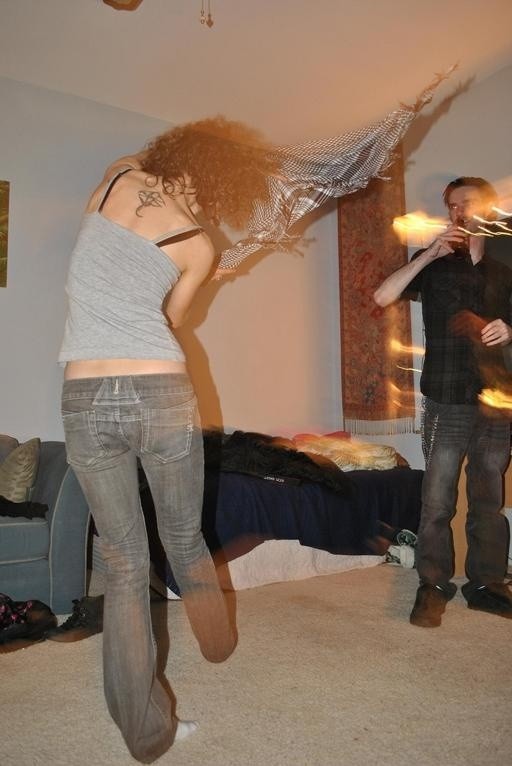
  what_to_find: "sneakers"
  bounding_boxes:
[463,582,512,618]
[47,595,104,643]
[410,581,456,629]
[0,594,58,655]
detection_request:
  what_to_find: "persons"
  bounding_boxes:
[372,178,512,628]
[54,114,269,765]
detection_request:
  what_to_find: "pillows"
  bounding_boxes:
[0,433,19,467]
[0,436,42,504]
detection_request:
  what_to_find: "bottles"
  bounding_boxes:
[452,217,469,258]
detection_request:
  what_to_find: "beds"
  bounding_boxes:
[88,428,425,601]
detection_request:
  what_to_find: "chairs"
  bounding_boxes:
[0,441,92,627]
